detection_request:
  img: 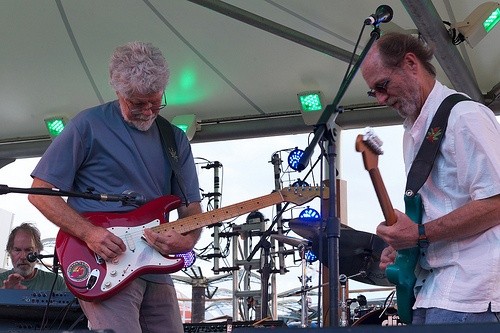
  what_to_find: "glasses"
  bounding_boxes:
[366,52,407,98]
[119,90,168,116]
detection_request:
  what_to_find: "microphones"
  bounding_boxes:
[364,5,393,25]
[92,189,146,206]
[318,141,339,177]
[27,252,37,263]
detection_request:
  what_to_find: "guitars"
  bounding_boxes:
[55,184,330,303]
[354,126,423,324]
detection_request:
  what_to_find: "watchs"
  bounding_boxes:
[416,225,431,249]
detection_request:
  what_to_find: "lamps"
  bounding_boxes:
[172,114,202,141]
[458,1,500,49]
[43,115,69,142]
[298,91,325,125]
[288,146,321,263]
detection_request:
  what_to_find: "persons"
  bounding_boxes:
[0,223,66,290]
[360,31,500,325]
[28,41,203,333]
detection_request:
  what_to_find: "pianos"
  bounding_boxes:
[1,283,91,333]
